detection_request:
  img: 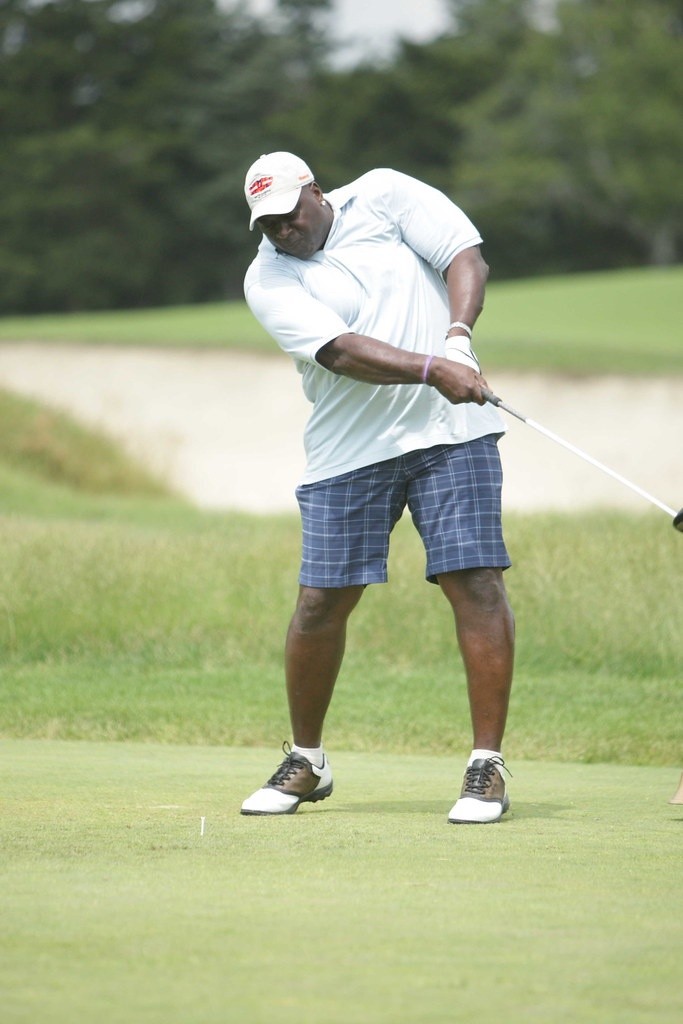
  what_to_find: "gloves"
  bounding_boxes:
[443,334,482,376]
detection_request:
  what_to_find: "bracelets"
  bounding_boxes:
[445,322,473,340]
[423,354,435,383]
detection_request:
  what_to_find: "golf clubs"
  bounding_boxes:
[483,387,682,535]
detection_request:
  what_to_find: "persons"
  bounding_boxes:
[241,150,510,822]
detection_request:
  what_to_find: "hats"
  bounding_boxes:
[244,151,315,233]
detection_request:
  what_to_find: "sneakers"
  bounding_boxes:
[445,757,514,824]
[238,740,334,817]
